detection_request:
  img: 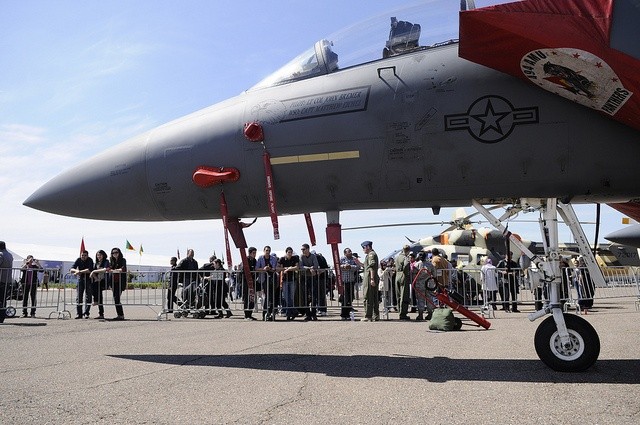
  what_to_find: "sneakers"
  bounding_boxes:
[31,314,36,318]
[361,317,372,321]
[20,313,27,318]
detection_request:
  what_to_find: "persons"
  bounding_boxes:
[561,256,594,310]
[19,254,45,318]
[431,248,450,306]
[395,245,411,320]
[497,251,521,313]
[378,261,391,312]
[352,241,380,321]
[166,256,183,315]
[199,255,218,315]
[104,248,126,321]
[407,253,417,312]
[237,247,258,321]
[175,249,196,310]
[276,247,299,321]
[0,240,13,322]
[70,251,93,319]
[326,270,336,300]
[387,259,398,312]
[226,264,242,302]
[480,259,497,309]
[415,252,436,320]
[41,271,49,291]
[338,248,363,321]
[310,250,329,316]
[298,243,318,321]
[255,246,280,320]
[204,259,233,319]
[89,250,109,319]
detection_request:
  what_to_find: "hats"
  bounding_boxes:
[361,241,372,247]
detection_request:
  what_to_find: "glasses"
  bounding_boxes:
[82,255,86,256]
[301,249,305,250]
[111,252,118,254]
[266,250,270,251]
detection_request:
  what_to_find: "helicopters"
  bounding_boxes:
[22,1,640,372]
[603,223,639,249]
[340,204,640,276]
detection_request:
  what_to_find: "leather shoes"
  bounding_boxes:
[94,315,104,319]
[75,314,82,319]
[214,312,223,318]
[225,311,232,318]
[85,314,89,318]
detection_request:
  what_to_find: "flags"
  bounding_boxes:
[126,239,136,251]
[80,240,87,258]
[138,243,144,256]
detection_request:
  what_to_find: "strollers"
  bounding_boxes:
[4,280,23,317]
[174,279,209,318]
